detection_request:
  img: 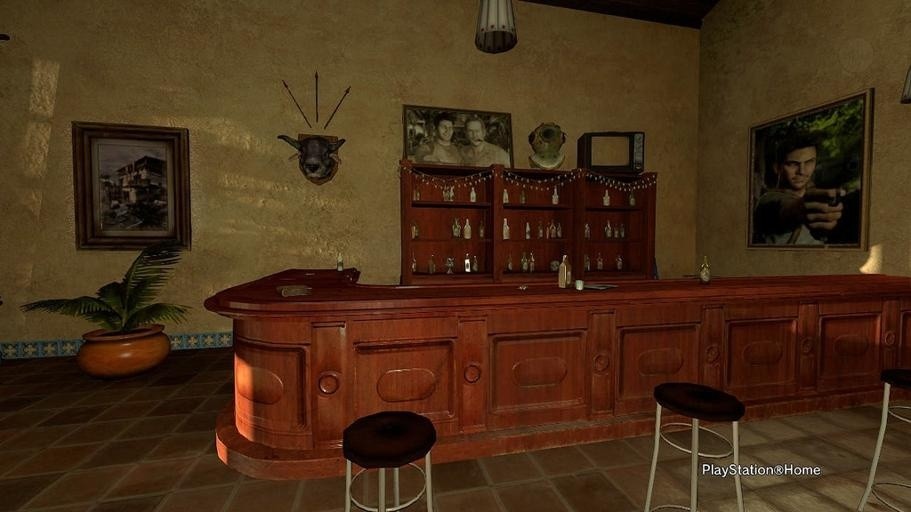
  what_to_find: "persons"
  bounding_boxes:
[460,115,511,167]
[414,112,462,164]
[751,132,847,245]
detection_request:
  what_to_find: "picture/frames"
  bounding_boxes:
[71,120,192,254]
[744,87,875,252]
[402,104,514,170]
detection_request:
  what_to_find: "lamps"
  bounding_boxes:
[900,63,911,104]
[475,0,519,54]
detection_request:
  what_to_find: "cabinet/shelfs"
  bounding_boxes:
[397,159,659,286]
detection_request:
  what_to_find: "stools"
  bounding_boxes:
[855,366,911,512]
[342,410,438,512]
[643,381,746,512]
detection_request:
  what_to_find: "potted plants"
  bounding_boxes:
[18,236,196,381]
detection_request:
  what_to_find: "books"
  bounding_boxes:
[477,138,484,142]
